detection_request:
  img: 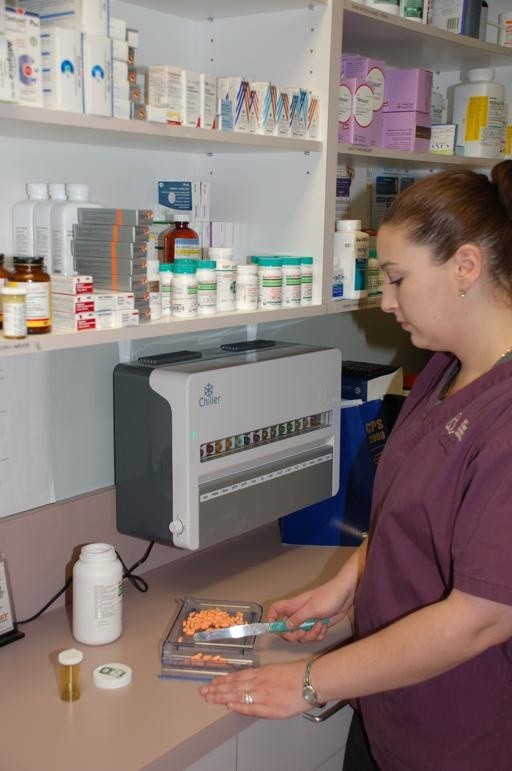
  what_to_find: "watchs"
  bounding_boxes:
[302,659,327,709]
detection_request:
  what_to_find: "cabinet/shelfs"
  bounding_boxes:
[238,668,357,769]
[0,0,510,358]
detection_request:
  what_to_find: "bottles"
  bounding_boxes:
[148,213,318,321]
[0,253,14,329]
[450,1,512,163]
[72,540,124,648]
[57,650,85,704]
[9,256,53,336]
[0,287,27,342]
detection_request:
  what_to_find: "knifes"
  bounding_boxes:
[193,618,328,647]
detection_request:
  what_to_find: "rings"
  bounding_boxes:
[245,691,252,704]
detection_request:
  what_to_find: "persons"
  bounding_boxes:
[199,161,512,771]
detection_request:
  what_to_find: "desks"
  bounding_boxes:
[0,516,368,769]
